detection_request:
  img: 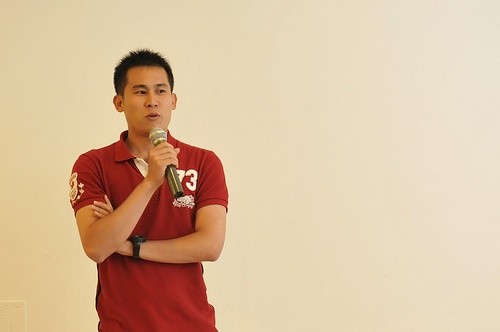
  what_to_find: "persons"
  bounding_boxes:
[69,50,228,332]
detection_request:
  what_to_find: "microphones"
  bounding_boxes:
[149,128,184,198]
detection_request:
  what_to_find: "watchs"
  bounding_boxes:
[130,236,147,259]
